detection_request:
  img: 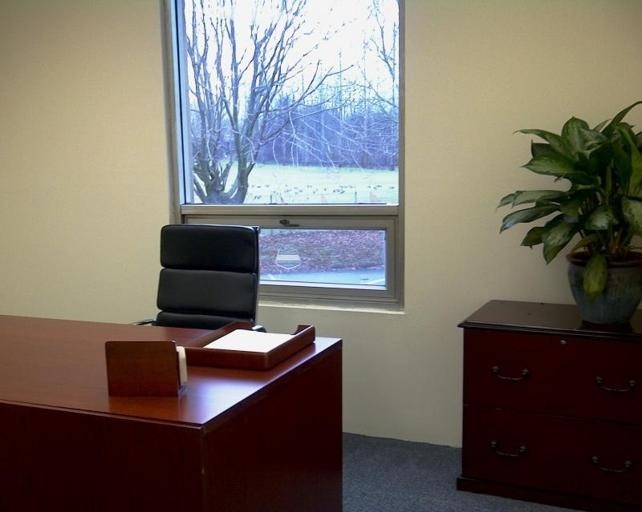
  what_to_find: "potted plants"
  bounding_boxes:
[496,101,642,325]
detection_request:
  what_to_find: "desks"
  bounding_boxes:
[0,315,344,511]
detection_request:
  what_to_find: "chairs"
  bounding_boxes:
[132,223,267,331]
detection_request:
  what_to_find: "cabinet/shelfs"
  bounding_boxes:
[456,300,642,511]
